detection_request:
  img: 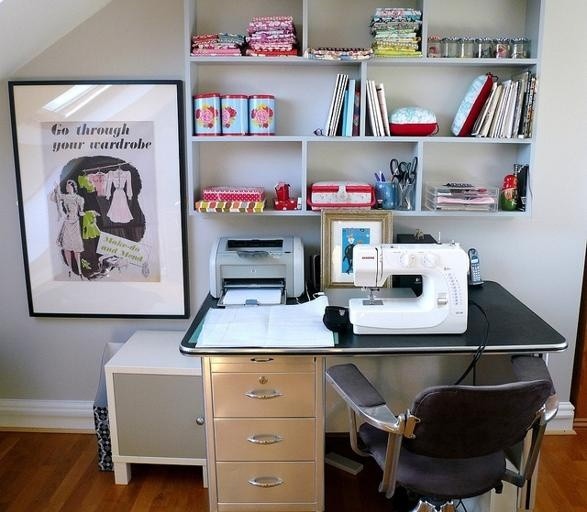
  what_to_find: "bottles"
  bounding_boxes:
[193,91,277,137]
[427,35,531,60]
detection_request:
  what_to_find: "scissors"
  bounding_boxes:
[390,156,417,209]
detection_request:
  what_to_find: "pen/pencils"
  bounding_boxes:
[374,170,386,182]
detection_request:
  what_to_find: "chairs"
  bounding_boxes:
[324,355,558,511]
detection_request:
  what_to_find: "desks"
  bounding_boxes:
[180,281,568,511]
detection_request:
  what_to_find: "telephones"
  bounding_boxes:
[468,248,484,286]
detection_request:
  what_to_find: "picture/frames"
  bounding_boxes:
[7,79,190,320]
[321,208,393,289]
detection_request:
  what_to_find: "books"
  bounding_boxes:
[474,71,536,139]
[195,186,267,213]
[324,72,390,138]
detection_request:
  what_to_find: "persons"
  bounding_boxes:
[52,179,85,281]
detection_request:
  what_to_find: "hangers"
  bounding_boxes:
[82,164,121,175]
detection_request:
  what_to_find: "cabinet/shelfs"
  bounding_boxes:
[183,0,546,218]
[104,329,208,488]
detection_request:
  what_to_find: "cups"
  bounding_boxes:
[395,183,416,212]
[374,181,395,210]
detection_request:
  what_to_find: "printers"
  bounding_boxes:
[208,234,304,306]
